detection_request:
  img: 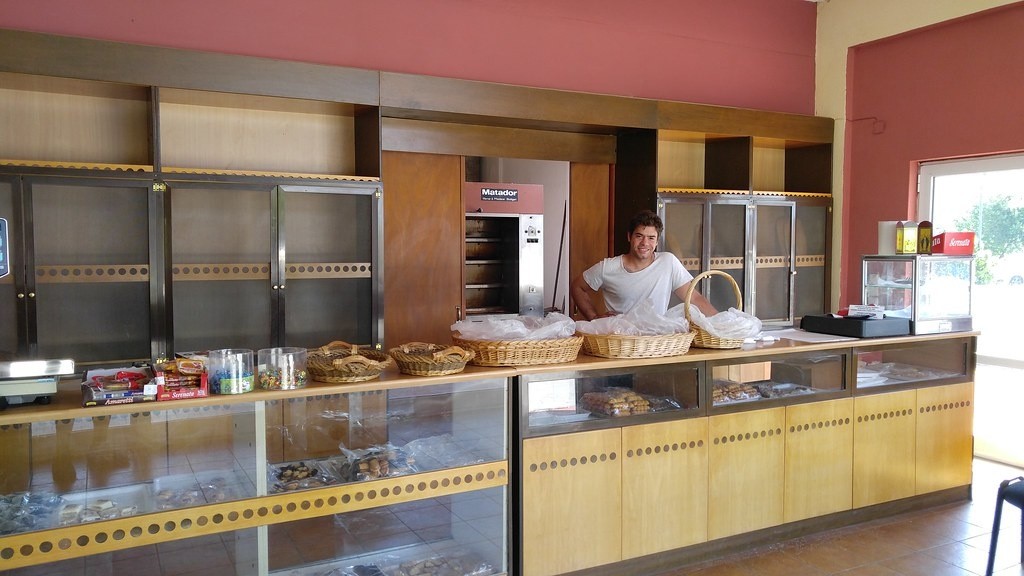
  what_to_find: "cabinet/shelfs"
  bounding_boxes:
[859,254,975,336]
[0,364,518,576]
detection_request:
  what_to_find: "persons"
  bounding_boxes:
[571,210,719,388]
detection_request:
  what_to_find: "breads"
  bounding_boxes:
[711,380,758,402]
[400,549,498,576]
[267,461,326,493]
[58,499,139,527]
[341,447,416,483]
[155,478,226,509]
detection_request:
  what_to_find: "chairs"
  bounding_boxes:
[984,476,1024,576]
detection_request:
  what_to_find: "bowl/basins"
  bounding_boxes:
[209,349,254,395]
[258,347,309,390]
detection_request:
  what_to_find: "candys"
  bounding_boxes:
[209,369,253,394]
[258,367,307,390]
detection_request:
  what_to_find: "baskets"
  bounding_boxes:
[451,329,585,368]
[307,341,392,383]
[575,330,699,359]
[684,270,745,349]
[389,341,476,376]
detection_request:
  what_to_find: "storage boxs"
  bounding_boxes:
[932,231,975,255]
[151,364,210,402]
[81,365,157,408]
[770,360,842,389]
